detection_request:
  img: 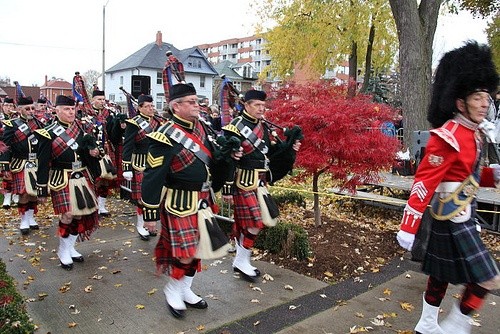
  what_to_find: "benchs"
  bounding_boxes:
[328,188,408,212]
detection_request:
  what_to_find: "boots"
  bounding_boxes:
[439,302,474,334]
[13,193,21,203]
[97,195,109,216]
[19,213,29,233]
[412,292,445,334]
[3,192,12,208]
[56,236,74,269]
[182,273,208,310]
[164,273,188,319]
[70,234,83,263]
[28,208,40,230]
[231,242,261,279]
[135,212,150,240]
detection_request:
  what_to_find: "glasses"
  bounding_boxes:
[23,106,35,112]
[4,104,14,108]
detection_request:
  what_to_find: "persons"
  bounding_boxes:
[397,90,500,334]
[368,114,403,139]
[34,95,99,270]
[140,83,244,318]
[0,89,220,239]
[223,90,301,281]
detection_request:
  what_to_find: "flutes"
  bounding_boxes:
[0,50,304,192]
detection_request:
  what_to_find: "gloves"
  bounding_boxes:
[396,230,417,251]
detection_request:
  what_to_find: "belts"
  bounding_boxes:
[434,182,463,192]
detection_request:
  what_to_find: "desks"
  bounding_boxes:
[347,170,500,235]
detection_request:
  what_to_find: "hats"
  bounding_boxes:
[92,90,105,98]
[137,94,154,104]
[17,96,33,106]
[244,89,266,103]
[4,97,14,104]
[425,41,498,129]
[36,97,47,104]
[54,94,75,110]
[166,83,196,99]
